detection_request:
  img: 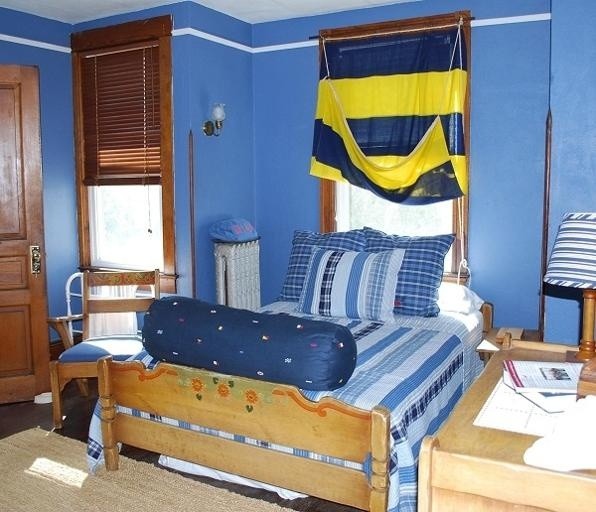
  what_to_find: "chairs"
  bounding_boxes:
[49,268,161,429]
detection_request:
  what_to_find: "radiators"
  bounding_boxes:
[211,238,263,311]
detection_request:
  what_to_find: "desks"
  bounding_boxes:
[418,330,596,512]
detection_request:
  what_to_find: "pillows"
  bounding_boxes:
[142,296,357,392]
[437,282,485,314]
[363,225,457,318]
[291,246,407,322]
[278,229,366,301]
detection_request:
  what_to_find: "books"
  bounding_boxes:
[502,359,585,413]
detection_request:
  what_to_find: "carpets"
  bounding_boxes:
[0,427,296,512]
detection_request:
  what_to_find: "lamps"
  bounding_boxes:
[541,212,596,363]
[204,102,227,136]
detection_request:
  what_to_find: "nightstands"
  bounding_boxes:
[475,328,540,366]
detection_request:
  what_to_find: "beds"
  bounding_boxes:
[85,302,493,512]
